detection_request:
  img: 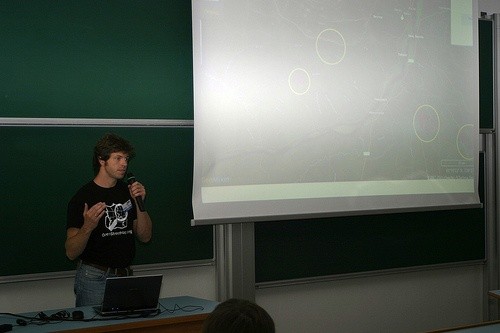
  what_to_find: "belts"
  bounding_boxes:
[81,260,133,276]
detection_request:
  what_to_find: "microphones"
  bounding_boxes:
[127,173,145,212]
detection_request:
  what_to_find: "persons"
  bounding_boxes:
[203,299,275,333]
[65,134,152,307]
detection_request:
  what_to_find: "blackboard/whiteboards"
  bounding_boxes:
[0,0,497,290]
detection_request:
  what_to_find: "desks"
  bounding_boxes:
[0,296,221,333]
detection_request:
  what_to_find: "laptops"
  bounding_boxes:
[92,274,163,317]
